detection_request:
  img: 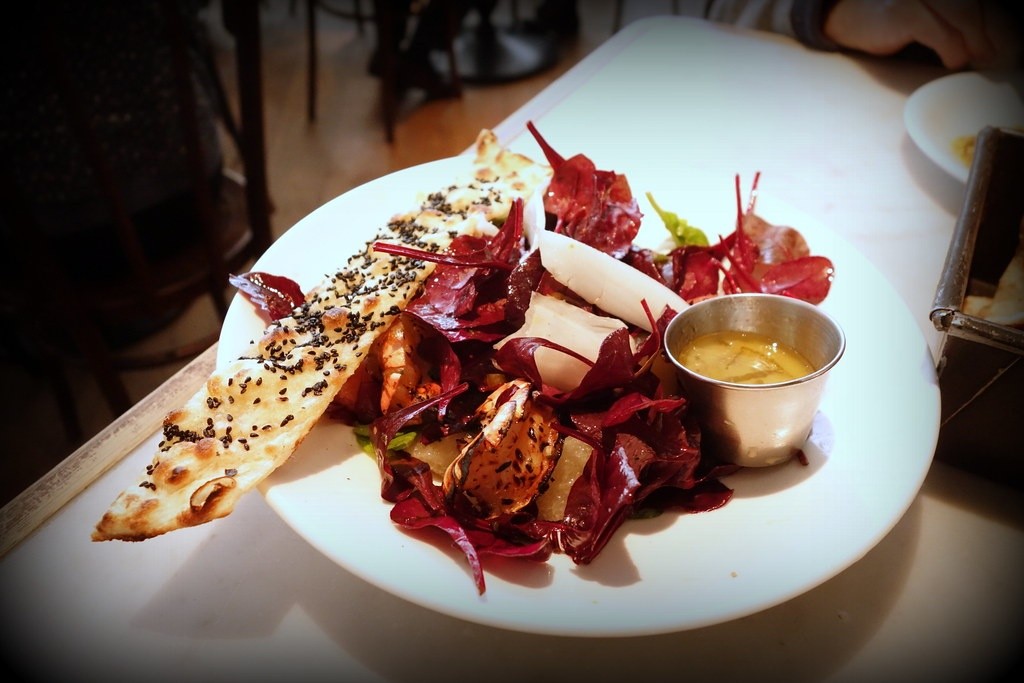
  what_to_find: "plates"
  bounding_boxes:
[213,154,943,640]
[901,72,1024,186]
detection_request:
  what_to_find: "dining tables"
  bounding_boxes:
[0,15,1024,683]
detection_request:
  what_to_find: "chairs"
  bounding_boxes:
[18,0,274,450]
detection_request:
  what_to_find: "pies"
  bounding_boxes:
[94,132,544,541]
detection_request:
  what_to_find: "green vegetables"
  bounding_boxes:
[646,192,707,246]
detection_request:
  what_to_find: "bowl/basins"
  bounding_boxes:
[662,291,848,470]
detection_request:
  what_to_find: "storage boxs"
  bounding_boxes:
[929,126,1024,504]
